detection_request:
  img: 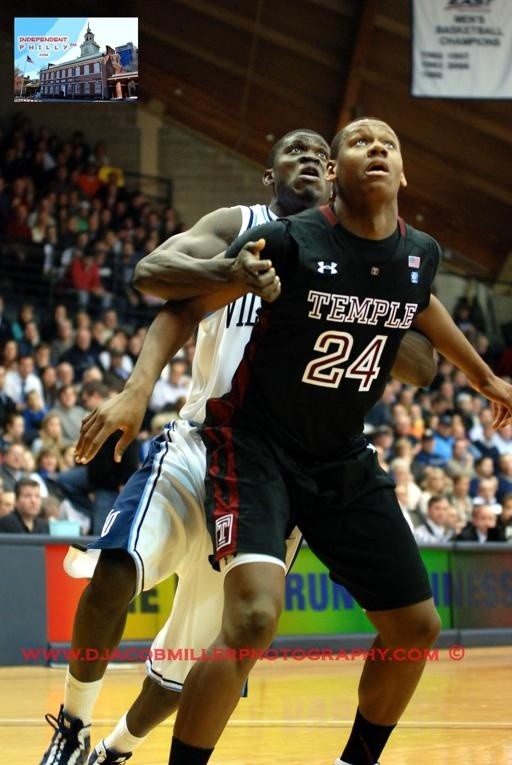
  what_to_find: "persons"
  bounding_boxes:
[0,111,512,543]
[36,129,439,765]
[72,116,512,765]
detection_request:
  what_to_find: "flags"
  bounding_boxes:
[26,56,32,63]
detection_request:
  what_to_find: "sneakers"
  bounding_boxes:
[39,704,90,765]
[90,739,133,765]
